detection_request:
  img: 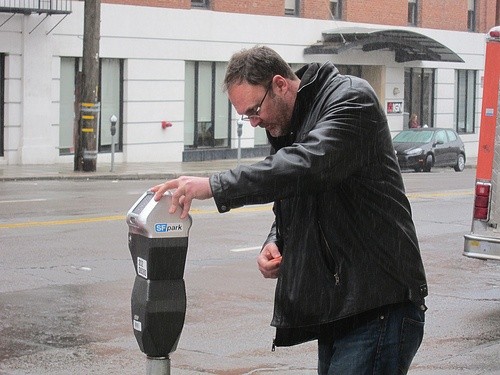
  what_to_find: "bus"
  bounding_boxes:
[460,28,500,265]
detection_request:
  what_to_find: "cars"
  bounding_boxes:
[390,128,466,171]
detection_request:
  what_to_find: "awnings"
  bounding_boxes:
[323,28,466,64]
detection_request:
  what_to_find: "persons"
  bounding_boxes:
[148,45,428,375]
[408,113,421,128]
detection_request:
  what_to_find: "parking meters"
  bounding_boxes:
[237,117,244,165]
[110,115,117,172]
[126,189,194,374]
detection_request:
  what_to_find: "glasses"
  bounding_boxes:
[241,81,272,121]
